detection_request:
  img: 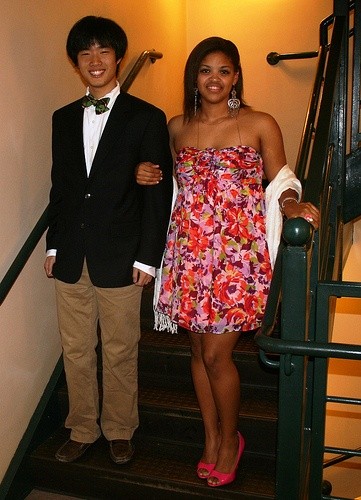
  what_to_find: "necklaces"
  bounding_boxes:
[202,119,226,125]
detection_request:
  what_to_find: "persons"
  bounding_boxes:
[44,15,174,468]
[136,36,319,487]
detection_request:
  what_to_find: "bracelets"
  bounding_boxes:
[281,197,298,208]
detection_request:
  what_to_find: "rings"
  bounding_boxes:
[305,214,313,222]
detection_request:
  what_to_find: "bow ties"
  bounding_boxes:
[81,92,110,115]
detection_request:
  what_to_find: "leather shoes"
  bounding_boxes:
[109,437,133,464]
[56,438,89,463]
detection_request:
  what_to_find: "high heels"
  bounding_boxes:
[197,432,221,479]
[207,431,245,488]
